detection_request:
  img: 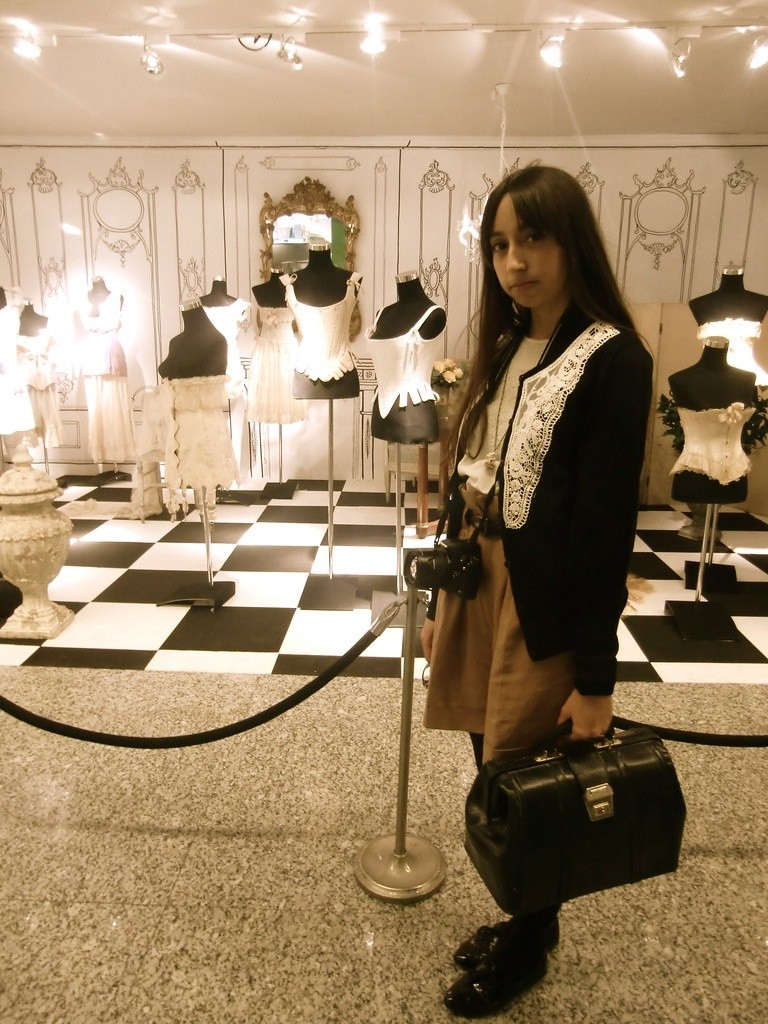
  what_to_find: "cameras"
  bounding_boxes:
[403,539,483,601]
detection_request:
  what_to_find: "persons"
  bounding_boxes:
[157,275,252,523]
[83,274,141,463]
[421,165,654,1019]
[667,264,768,504]
[364,270,447,445]
[0,285,82,450]
[245,238,364,424]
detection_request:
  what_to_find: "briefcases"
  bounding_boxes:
[464,716,687,918]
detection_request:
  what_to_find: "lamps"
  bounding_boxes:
[748,35,768,70]
[276,36,302,71]
[538,27,566,67]
[141,34,164,76]
[670,37,691,78]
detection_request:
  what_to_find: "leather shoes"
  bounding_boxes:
[439,917,563,1021]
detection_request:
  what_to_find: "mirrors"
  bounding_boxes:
[256,176,362,343]
